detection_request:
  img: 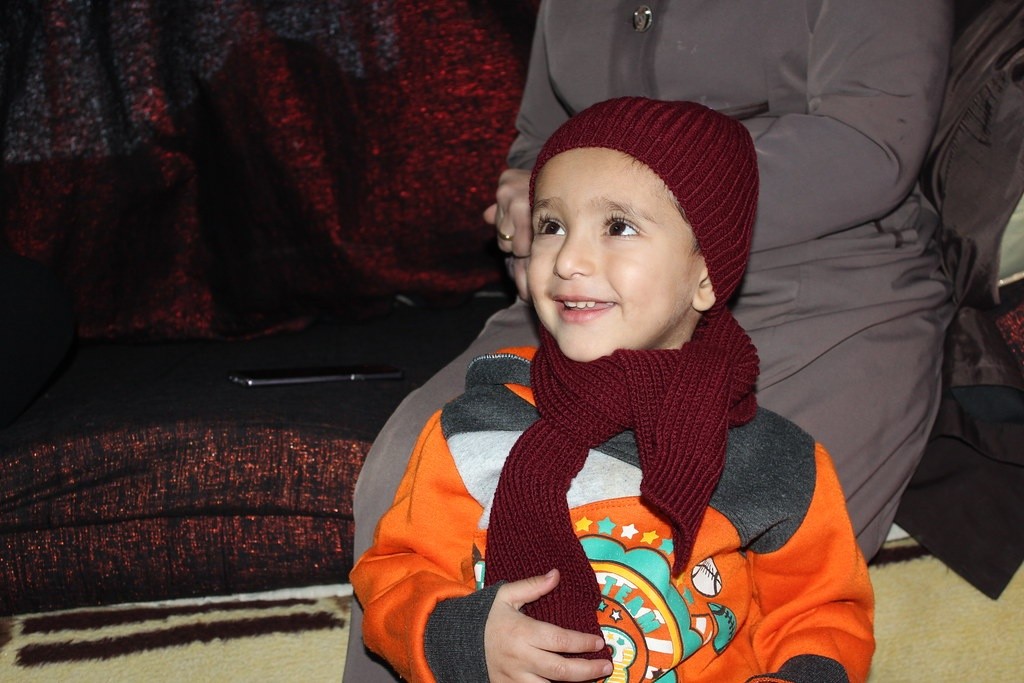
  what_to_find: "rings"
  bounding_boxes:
[496,229,513,241]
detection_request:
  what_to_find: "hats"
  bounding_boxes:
[528,96,759,317]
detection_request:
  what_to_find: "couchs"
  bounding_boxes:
[0,0,1023,682]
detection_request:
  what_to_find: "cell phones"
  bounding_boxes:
[227,365,403,385]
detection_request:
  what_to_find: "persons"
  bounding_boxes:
[342,0,950,683]
[350,96,874,683]
